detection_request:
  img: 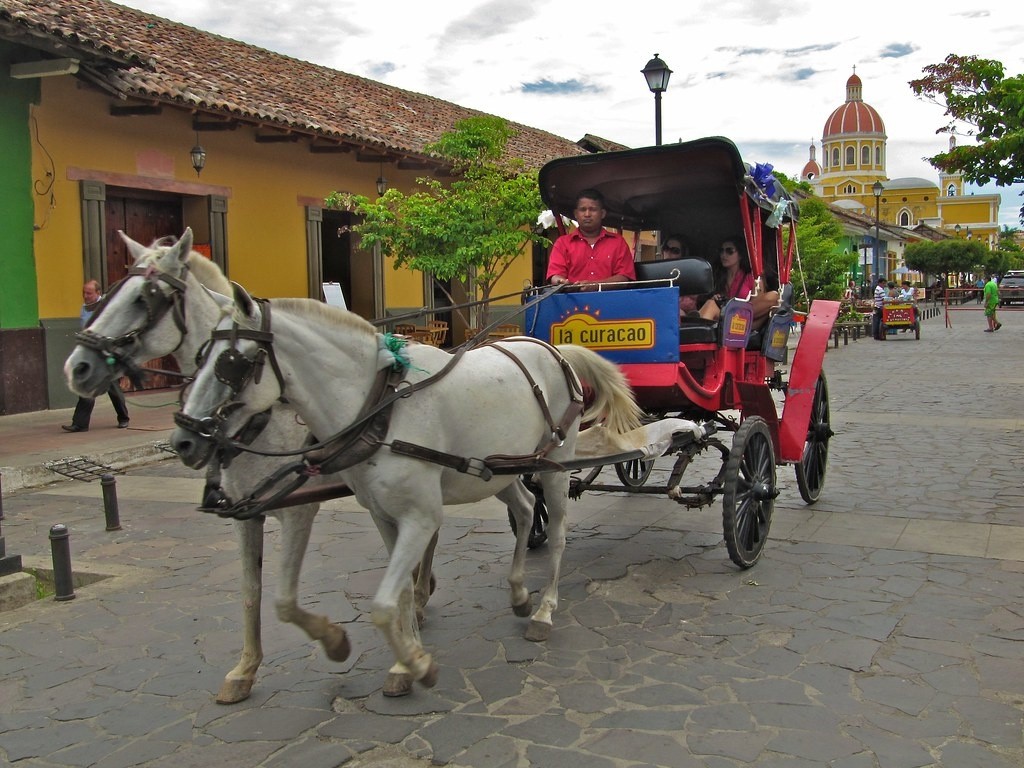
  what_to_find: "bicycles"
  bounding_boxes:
[977,286,983,305]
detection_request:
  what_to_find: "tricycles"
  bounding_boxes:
[878,295,922,341]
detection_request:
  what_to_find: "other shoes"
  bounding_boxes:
[746,333,761,351]
[995,323,1001,330]
[984,329,993,332]
[686,310,700,318]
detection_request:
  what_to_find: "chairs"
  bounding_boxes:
[428,321,447,344]
[496,324,520,340]
[395,324,416,342]
[464,328,481,340]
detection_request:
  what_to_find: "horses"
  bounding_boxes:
[61,226,646,705]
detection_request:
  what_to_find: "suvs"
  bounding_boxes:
[997,270,1024,308]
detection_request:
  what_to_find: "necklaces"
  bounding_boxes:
[726,283,732,290]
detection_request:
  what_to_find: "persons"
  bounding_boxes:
[61,281,131,431]
[657,234,779,347]
[842,274,1002,341]
[546,189,636,292]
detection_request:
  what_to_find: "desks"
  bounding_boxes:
[488,331,522,338]
[416,327,449,347]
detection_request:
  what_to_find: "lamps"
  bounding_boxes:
[190,111,205,178]
[376,157,386,196]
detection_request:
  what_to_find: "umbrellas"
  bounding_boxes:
[892,265,918,283]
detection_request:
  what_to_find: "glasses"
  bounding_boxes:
[662,245,682,255]
[718,247,738,255]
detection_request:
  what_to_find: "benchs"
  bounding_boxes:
[747,276,779,350]
[635,257,716,352]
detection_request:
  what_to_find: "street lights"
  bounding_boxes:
[871,180,884,283]
[640,52,676,145]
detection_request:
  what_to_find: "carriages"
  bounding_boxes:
[63,137,842,707]
